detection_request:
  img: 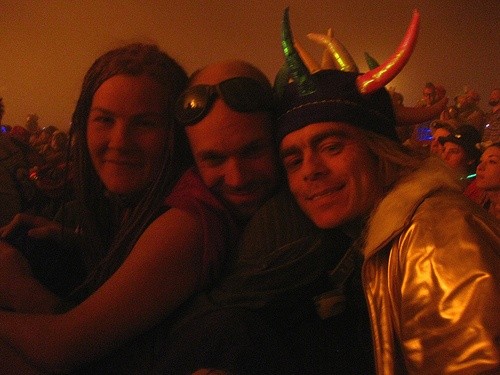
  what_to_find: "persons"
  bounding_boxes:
[0,44,499,375]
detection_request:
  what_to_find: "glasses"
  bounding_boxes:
[173,77,270,124]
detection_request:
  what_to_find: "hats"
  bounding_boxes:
[437,124,482,160]
[272,70,401,139]
